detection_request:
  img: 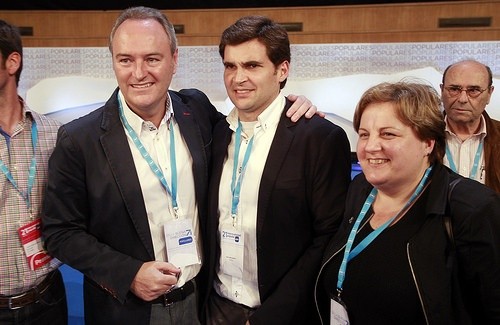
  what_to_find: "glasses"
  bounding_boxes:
[443,85,490,98]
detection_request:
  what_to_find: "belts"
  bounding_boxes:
[0,272,54,310]
[151,278,196,307]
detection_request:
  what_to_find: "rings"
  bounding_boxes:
[165,285,176,295]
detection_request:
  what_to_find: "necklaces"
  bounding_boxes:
[444,138,484,181]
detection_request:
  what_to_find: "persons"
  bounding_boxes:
[435,60,500,198]
[41,7,325,325]
[200,17,352,325]
[0,18,69,325]
[321,84,500,325]
